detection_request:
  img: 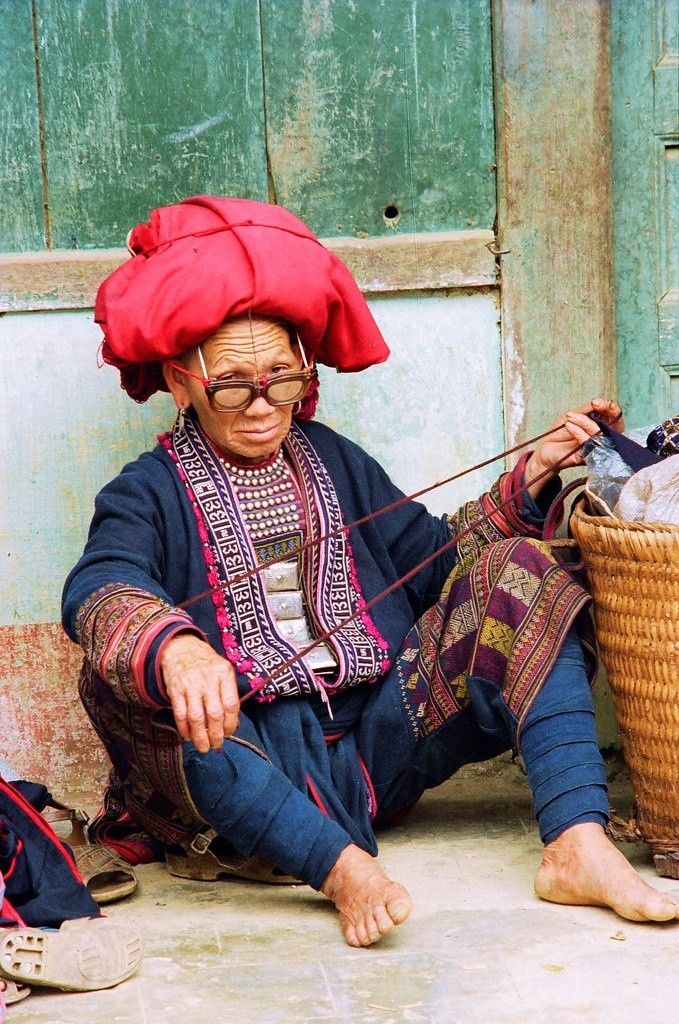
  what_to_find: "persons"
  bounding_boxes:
[62,194,679,948]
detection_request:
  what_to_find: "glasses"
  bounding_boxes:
[195,330,316,413]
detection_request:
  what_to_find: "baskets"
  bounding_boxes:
[569,487,679,881]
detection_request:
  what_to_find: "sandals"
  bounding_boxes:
[0,978,30,1003]
[164,827,308,884]
[39,797,138,902]
[0,914,143,991]
